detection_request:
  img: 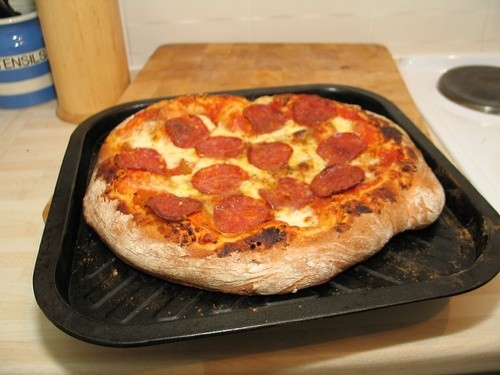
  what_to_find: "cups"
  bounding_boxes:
[0,0,54,109]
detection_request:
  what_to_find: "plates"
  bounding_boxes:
[32,83,499,347]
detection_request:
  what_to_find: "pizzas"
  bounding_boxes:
[82,92,446,296]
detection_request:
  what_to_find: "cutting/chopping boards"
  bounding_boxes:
[42,42,429,224]
